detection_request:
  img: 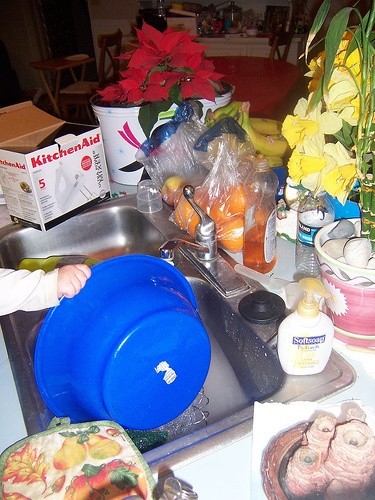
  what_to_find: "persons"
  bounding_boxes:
[0,264,92,318]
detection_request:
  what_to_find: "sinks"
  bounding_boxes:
[0,198,181,277]
[10,268,356,461]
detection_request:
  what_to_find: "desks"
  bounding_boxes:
[31,57,94,119]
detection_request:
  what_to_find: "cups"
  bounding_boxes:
[136,179,162,214]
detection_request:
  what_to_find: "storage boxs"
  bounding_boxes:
[0,101,113,232]
[166,10,197,34]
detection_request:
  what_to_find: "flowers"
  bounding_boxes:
[280,0,375,244]
[99,19,227,138]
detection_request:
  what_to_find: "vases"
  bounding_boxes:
[90,84,234,185]
[314,218,375,349]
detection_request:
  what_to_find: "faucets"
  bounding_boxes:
[159,183,218,262]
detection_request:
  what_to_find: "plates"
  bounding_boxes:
[0,419,164,499]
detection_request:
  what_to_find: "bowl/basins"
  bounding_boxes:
[34,254,211,431]
[246,29,258,36]
[225,28,239,33]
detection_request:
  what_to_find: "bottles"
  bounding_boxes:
[223,1,243,26]
[157,0,165,17]
[196,13,202,35]
[242,153,279,275]
[295,189,335,278]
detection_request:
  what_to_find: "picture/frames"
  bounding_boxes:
[262,5,288,33]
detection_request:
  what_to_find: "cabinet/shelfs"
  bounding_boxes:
[199,36,301,65]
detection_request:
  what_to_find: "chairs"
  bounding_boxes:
[60,27,122,124]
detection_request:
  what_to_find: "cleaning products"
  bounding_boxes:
[275,276,336,376]
[242,152,278,275]
